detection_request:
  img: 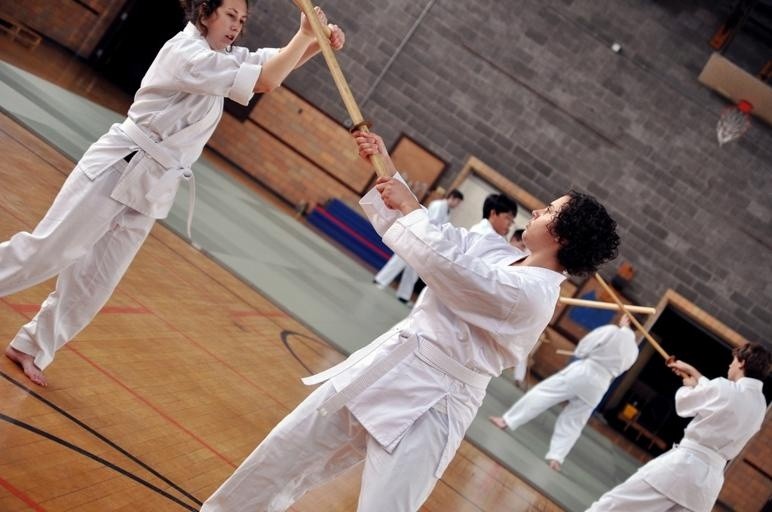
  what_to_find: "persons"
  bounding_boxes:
[413,192,519,309]
[509,229,526,251]
[370,189,464,305]
[580,342,772,512]
[199,128,619,512]
[511,357,530,388]
[1,0,347,389]
[487,309,648,472]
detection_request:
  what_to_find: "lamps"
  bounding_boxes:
[698,49,772,148]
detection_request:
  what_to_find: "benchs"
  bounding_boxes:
[0,14,42,50]
